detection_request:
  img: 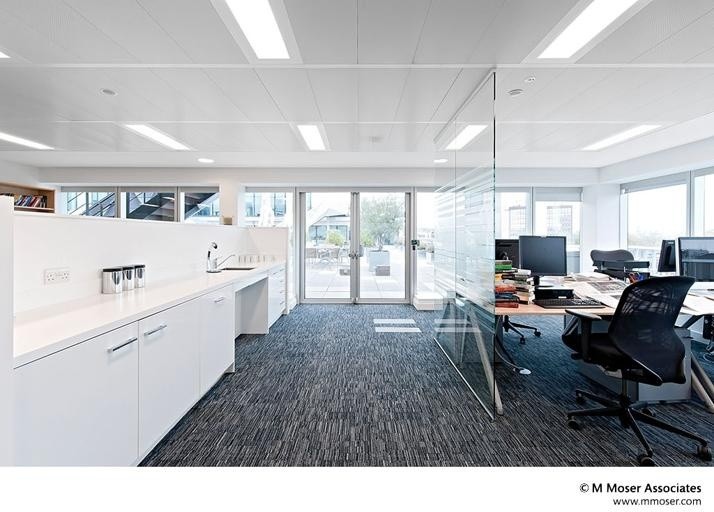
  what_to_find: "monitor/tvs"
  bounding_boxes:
[518,235,567,287]
[658,239,676,272]
[495,239,520,269]
[675,236,714,290]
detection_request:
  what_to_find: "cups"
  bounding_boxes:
[102,264,145,293]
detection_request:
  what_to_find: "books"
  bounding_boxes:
[476,259,536,309]
[0,193,48,208]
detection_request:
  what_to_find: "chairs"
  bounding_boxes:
[566,276,712,466]
[589,250,634,277]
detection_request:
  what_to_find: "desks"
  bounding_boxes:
[456,295,616,416]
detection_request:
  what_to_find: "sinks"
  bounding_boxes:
[219,268,255,270]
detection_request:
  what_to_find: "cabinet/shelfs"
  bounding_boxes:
[14,262,287,467]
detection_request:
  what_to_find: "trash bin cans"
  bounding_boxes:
[338,247,350,275]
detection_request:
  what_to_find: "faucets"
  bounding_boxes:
[212,254,236,268]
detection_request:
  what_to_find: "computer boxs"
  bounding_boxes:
[533,287,575,299]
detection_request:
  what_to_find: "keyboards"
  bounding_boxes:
[531,298,606,309]
